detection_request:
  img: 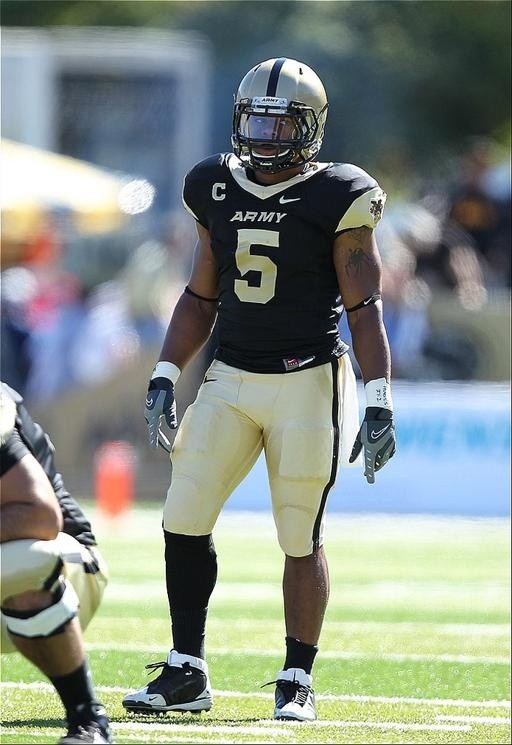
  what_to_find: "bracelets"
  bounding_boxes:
[361,374,392,412]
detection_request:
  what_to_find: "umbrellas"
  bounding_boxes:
[0,133,150,249]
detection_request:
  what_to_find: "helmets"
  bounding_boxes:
[234,57,329,174]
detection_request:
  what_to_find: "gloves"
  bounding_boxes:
[349,406,397,484]
[144,377,178,454]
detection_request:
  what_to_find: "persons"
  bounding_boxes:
[121,55,397,723]
[1,382,116,744]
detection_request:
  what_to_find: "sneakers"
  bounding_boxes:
[274,668,318,722]
[60,703,111,744]
[122,650,214,711]
